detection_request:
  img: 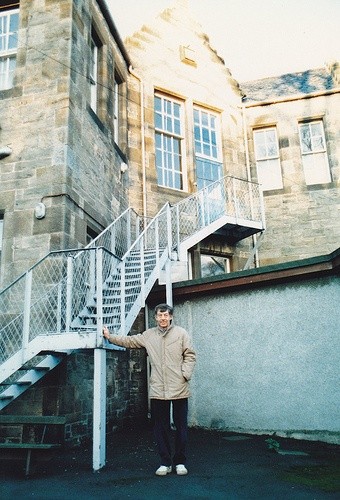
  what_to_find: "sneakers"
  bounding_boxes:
[155,465,172,476]
[175,464,187,476]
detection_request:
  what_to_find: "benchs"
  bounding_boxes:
[0,415,68,481]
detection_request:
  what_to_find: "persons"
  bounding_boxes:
[104,304,196,475]
